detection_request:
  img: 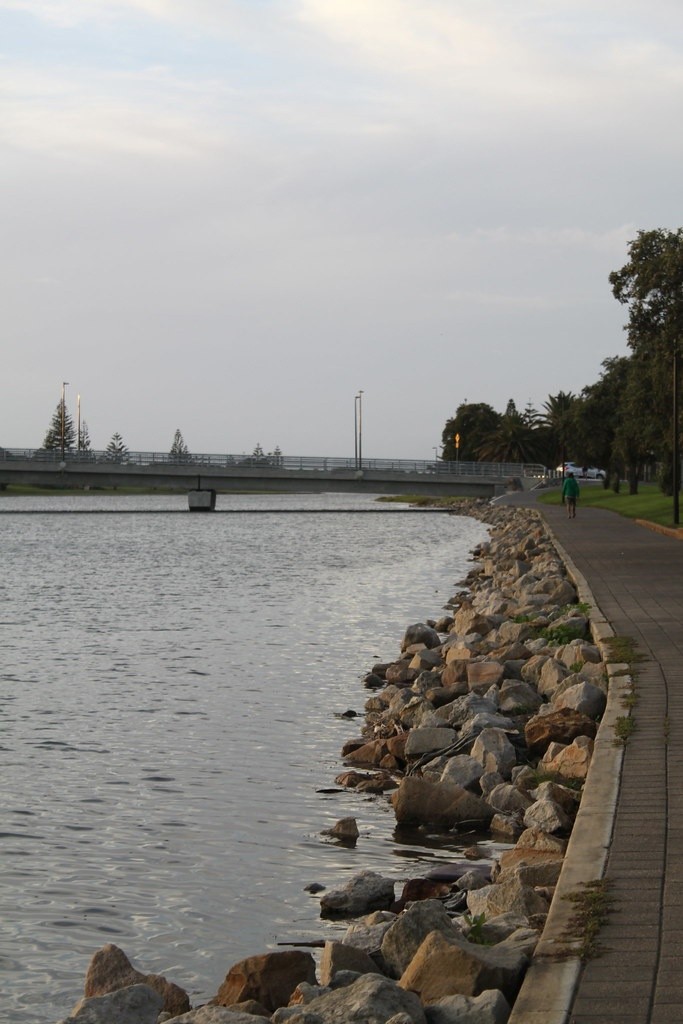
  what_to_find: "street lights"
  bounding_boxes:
[59,379,84,463]
[454,434,461,476]
[352,388,366,470]
[672,330,683,524]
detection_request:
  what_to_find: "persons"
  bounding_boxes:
[582,465,588,480]
[561,471,580,519]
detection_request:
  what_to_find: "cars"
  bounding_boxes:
[556,461,607,479]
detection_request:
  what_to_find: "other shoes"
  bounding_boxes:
[569,516,571,519]
[572,514,575,518]
